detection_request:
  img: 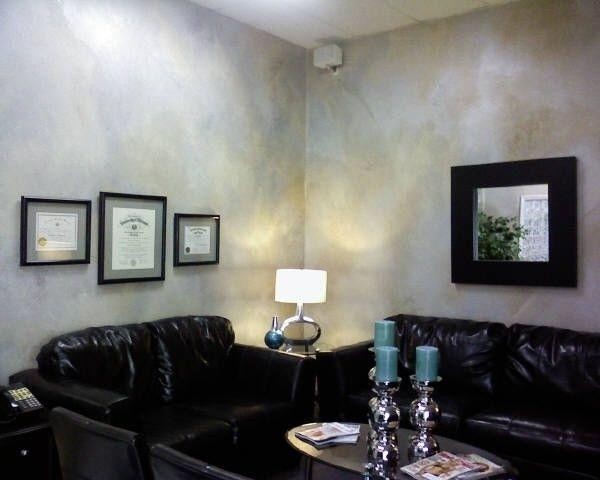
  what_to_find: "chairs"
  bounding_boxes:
[149,443,253,479]
[49,406,149,480]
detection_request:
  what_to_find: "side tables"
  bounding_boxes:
[1,410,64,480]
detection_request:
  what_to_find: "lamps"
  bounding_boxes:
[273,268,327,357]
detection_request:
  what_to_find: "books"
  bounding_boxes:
[400,451,505,480]
[295,422,360,449]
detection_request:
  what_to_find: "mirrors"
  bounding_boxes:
[451,155,578,288]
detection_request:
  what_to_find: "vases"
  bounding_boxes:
[265,316,284,349]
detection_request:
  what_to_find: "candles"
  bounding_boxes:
[415,345,439,382]
[373,320,396,349]
[374,345,399,382]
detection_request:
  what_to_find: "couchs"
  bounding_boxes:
[9,315,312,480]
[313,314,600,480]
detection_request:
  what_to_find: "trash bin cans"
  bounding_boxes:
[0,385,43,429]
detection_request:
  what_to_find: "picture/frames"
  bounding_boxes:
[21,196,91,265]
[98,192,165,284]
[173,213,221,267]
